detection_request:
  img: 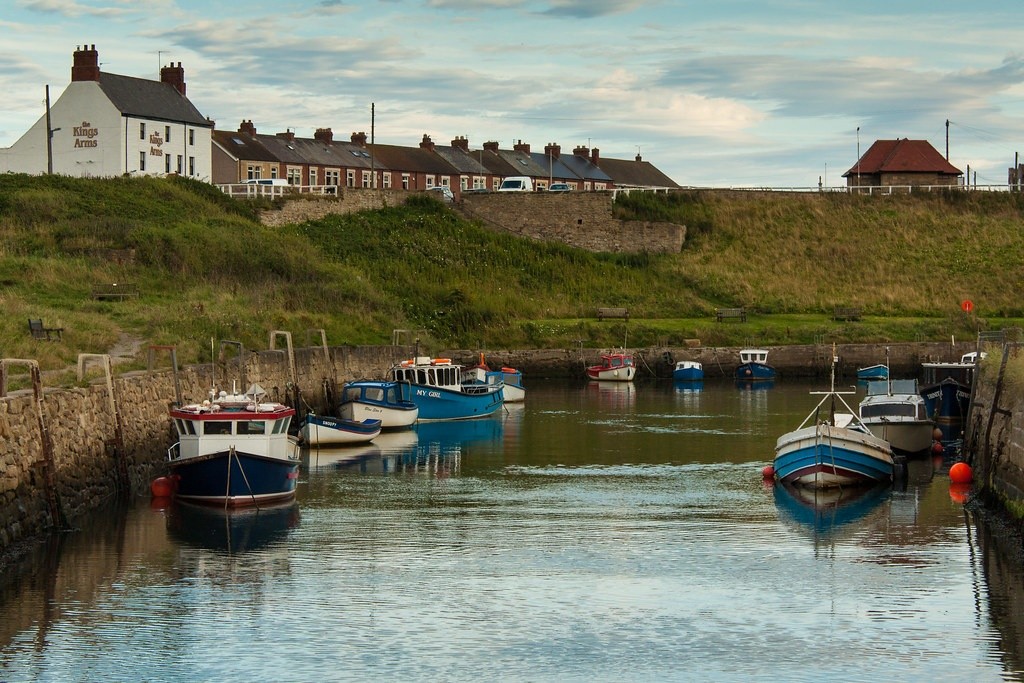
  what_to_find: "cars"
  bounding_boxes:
[549,183,569,192]
[425,186,454,204]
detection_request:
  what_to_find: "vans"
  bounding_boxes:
[239,179,288,186]
[498,176,533,192]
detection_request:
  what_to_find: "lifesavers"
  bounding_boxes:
[502,367,516,372]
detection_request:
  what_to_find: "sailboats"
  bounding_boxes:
[770,480,892,553]
[774,344,896,486]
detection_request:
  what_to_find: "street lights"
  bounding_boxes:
[857,127,861,194]
[825,162,827,192]
[45,84,62,175]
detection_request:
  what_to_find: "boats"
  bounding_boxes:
[588,381,637,402]
[586,353,636,381]
[166,507,300,554]
[390,357,505,422]
[672,381,704,396]
[857,364,890,381]
[920,377,971,441]
[734,349,776,381]
[461,353,495,384]
[672,360,704,381]
[301,445,381,472]
[165,394,302,506]
[856,348,936,459]
[337,379,419,428]
[485,367,526,402]
[962,352,988,364]
[918,362,975,392]
[300,412,382,444]
